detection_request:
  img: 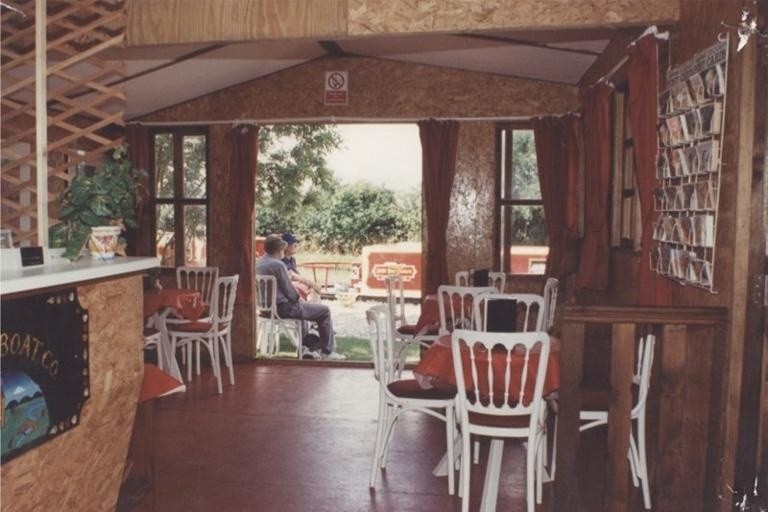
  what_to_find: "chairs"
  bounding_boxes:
[144,266,239,394]
[366,272,656,512]
[256,275,313,360]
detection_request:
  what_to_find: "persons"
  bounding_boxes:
[281,233,337,339]
[255,233,347,361]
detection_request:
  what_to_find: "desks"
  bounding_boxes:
[549,246,733,512]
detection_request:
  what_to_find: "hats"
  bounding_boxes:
[282,233,302,245]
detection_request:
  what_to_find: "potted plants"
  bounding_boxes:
[48,142,152,265]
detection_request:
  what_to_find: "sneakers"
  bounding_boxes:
[301,348,321,360]
[321,349,350,362]
